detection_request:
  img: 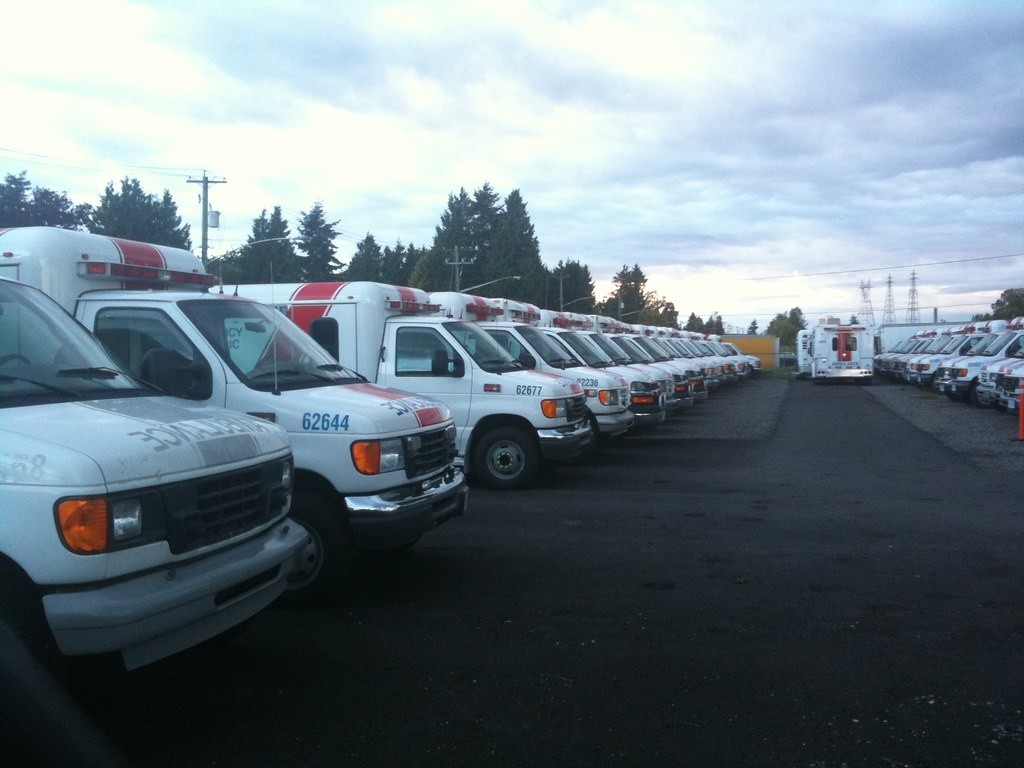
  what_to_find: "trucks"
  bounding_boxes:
[797,324,874,384]
[874,317,1023,408]
[0,278,308,680]
[427,291,761,436]
[199,281,594,489]
[0,226,469,607]
